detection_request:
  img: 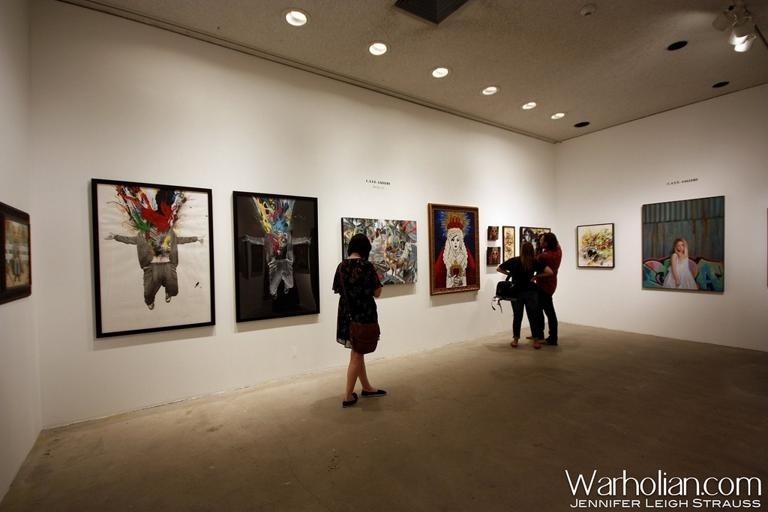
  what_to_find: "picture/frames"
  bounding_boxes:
[501,226,515,263]
[88,178,216,339]
[427,201,480,297]
[0,202,31,305]
[231,190,321,323]
[518,225,552,256]
[575,223,615,268]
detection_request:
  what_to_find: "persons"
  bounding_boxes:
[526,232,562,346]
[332,234,388,408]
[497,242,554,350]
[435,215,476,288]
[104,226,204,310]
[240,231,311,301]
[534,233,545,255]
[10,250,24,283]
[522,229,535,242]
[662,238,698,289]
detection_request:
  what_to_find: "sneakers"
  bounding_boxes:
[342,392,359,408]
[532,342,542,350]
[510,341,518,348]
[361,389,388,397]
[526,335,533,340]
[539,338,558,346]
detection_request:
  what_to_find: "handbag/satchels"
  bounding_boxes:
[495,272,523,303]
[348,321,381,355]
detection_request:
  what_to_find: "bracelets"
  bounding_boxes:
[538,274,541,279]
[541,274,544,280]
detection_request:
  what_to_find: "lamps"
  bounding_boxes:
[712,2,759,53]
